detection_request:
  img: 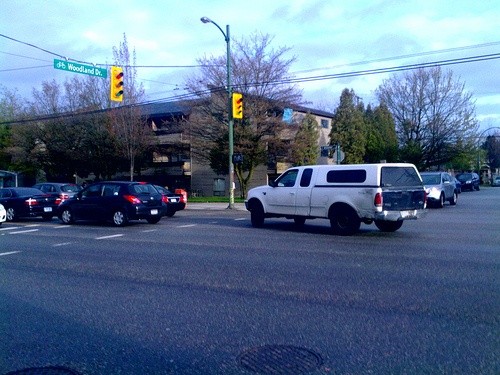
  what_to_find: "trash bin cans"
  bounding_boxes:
[213,177,225,196]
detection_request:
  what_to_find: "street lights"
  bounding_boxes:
[201,16,236,210]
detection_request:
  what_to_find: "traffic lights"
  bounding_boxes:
[232,92,243,118]
[110,66,124,102]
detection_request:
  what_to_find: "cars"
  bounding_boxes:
[153,184,186,218]
[32,183,82,202]
[455,173,480,192]
[57,181,167,227]
[0,203,7,226]
[0,187,62,221]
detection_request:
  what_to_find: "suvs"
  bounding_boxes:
[419,172,457,208]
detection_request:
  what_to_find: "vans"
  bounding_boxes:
[243,162,428,236]
